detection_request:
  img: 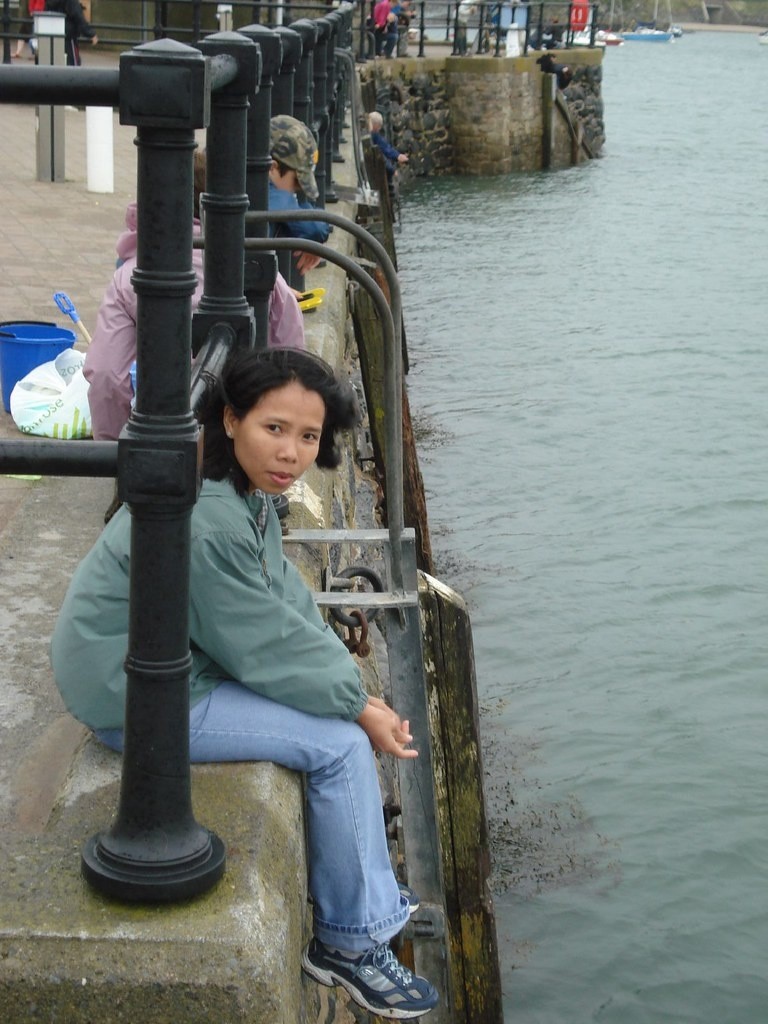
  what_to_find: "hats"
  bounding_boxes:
[269,115,319,198]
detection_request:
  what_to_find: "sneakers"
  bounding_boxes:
[301,936,439,1019]
[398,882,418,913]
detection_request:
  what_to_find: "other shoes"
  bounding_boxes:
[28,54,36,61]
[10,53,22,58]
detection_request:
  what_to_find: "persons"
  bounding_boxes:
[269,113,329,275]
[477,6,491,54]
[11,0,97,65]
[531,18,564,49]
[367,0,416,58]
[536,53,571,87]
[452,0,479,55]
[368,111,409,176]
[50,349,439,1019]
[85,151,304,442]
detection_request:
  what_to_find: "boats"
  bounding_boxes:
[593,29,623,46]
[620,30,673,44]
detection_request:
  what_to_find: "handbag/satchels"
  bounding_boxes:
[10,348,91,439]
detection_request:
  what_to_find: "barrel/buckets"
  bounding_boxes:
[0,321,75,412]
